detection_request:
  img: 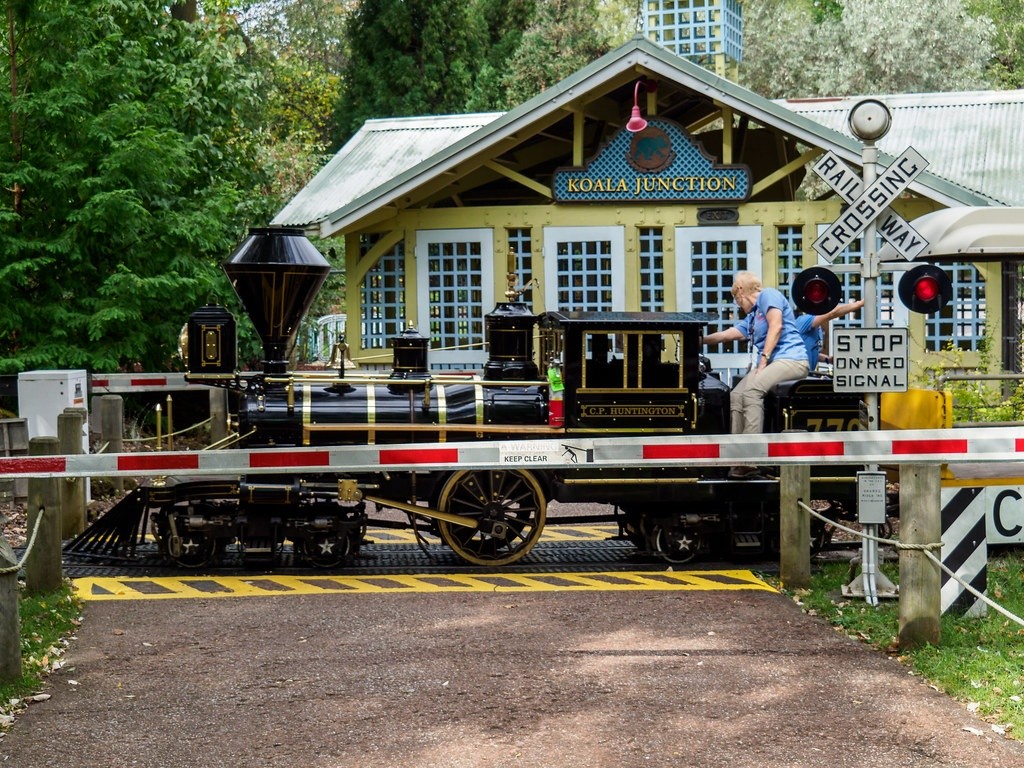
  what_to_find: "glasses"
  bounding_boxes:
[732,293,741,305]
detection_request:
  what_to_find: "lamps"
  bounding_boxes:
[626,78,655,132]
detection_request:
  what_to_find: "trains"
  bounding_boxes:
[144,227,1024,570]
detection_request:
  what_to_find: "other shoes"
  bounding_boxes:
[728,464,760,479]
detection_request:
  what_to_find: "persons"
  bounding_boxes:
[795,299,864,372]
[699,272,811,478]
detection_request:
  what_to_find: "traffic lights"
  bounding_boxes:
[791,266,842,316]
[898,264,953,314]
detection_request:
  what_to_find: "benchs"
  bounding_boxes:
[732,370,863,400]
